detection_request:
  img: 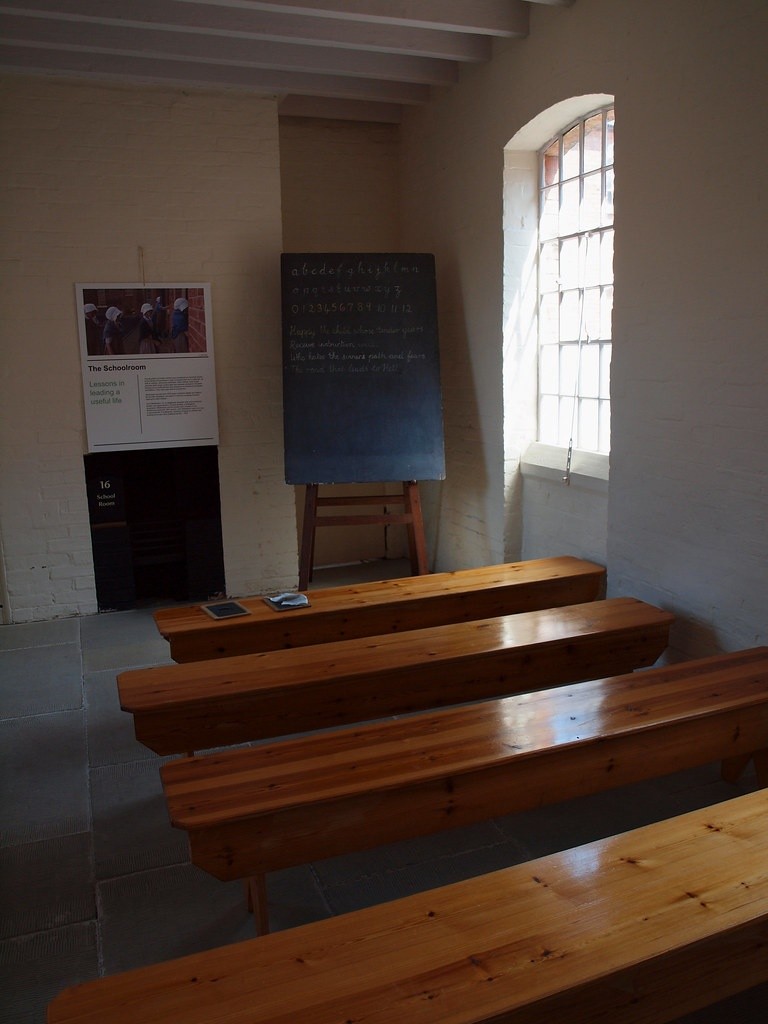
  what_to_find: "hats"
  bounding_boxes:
[156,297,161,304]
[84,304,97,314]
[106,307,122,321]
[141,303,154,315]
[174,298,188,311]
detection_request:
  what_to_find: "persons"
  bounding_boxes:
[84,297,189,356]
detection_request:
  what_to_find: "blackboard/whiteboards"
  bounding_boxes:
[283,252,447,483]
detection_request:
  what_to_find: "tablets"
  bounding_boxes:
[264,599,311,612]
[202,600,251,620]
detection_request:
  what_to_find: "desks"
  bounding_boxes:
[160,646,768,937]
[153,556,608,666]
[115,596,677,758]
[46,789,768,1024]
